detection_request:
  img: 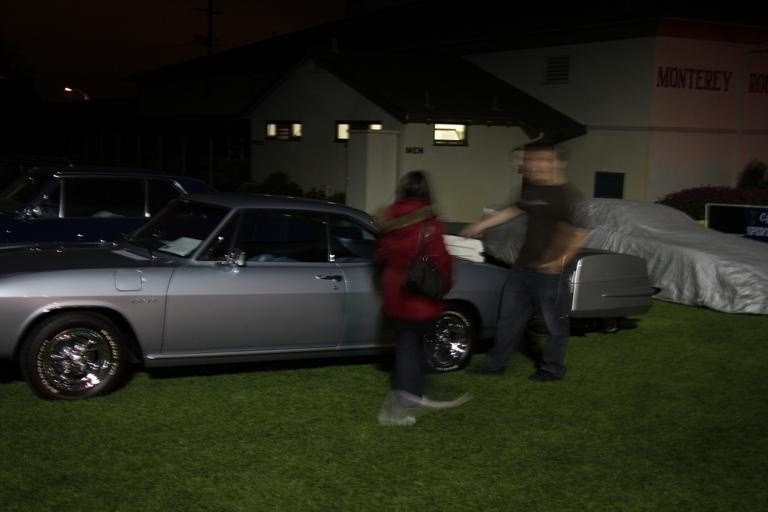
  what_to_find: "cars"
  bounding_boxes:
[2,196,518,399]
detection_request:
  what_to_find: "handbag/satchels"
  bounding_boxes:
[406,221,444,300]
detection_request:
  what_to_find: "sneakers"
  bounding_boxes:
[378,396,429,429]
[470,360,504,373]
[528,369,560,381]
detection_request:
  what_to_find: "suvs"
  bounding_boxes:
[1,167,218,245]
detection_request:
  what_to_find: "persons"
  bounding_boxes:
[480,144,527,264]
[374,170,452,405]
